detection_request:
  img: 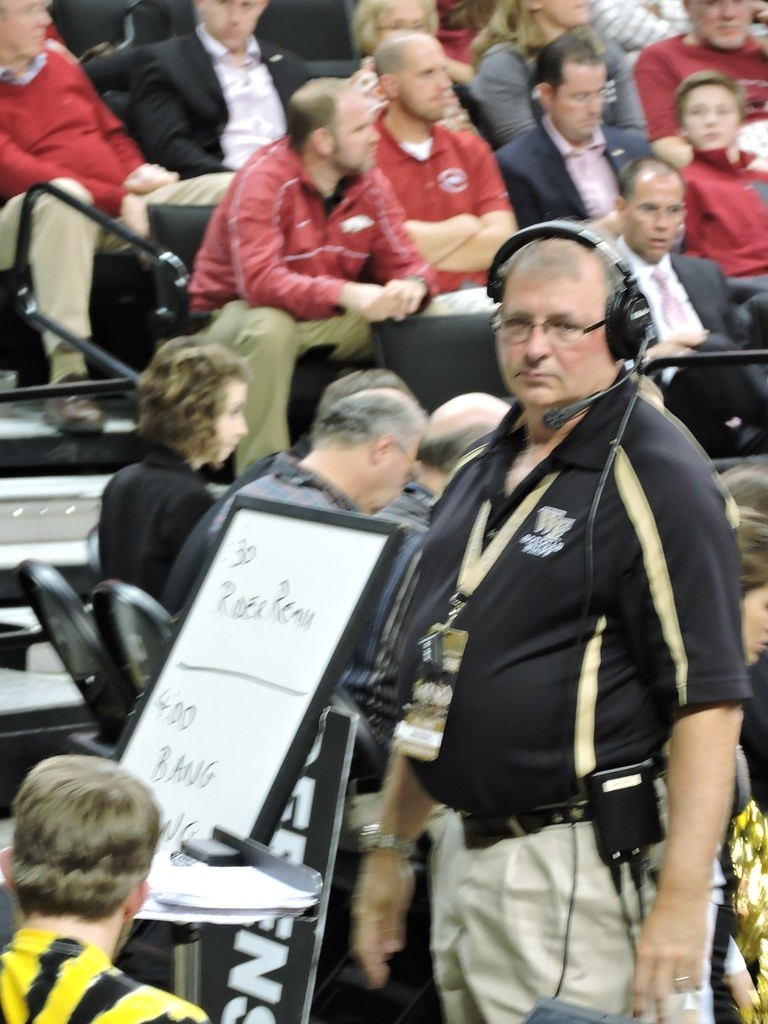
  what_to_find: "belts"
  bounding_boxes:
[457,798,590,835]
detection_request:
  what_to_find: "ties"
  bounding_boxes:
[652,272,686,331]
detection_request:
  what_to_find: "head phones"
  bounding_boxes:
[487,220,658,360]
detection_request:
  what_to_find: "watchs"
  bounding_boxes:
[357,822,416,857]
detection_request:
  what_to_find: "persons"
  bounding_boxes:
[369,29,521,317]
[469,0,648,151]
[162,366,429,614]
[0,0,243,431]
[634,0,767,169]
[186,75,441,481]
[673,68,767,353]
[432,0,494,64]
[586,0,694,138]
[493,35,686,248]
[1,753,210,1024]
[608,156,767,458]
[353,217,757,1024]
[347,0,440,69]
[326,391,514,860]
[96,346,250,590]
[133,0,313,183]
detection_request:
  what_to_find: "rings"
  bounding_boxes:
[673,976,692,982]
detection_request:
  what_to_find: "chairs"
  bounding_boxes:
[47,0,362,350]
[15,560,452,850]
[370,312,518,412]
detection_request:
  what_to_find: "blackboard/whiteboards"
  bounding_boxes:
[114,495,407,854]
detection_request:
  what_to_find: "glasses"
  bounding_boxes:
[488,305,605,345]
[573,88,610,104]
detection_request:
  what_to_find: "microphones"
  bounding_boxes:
[543,338,650,431]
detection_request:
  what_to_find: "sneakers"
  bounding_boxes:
[42,374,107,435]
